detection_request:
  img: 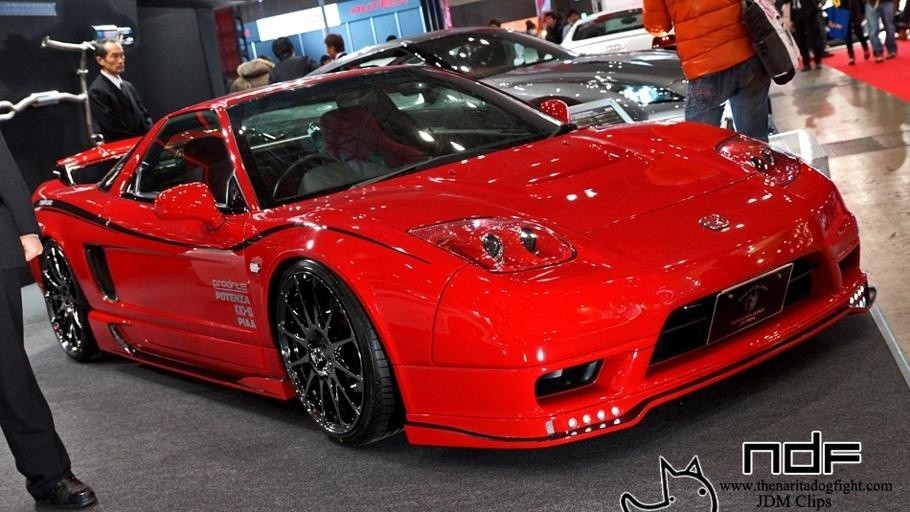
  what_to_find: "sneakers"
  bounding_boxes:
[801,50,898,73]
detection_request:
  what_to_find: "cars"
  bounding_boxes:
[303,26,739,135]
[558,5,677,56]
[822,1,884,47]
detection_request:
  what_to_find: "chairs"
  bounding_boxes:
[316,103,431,168]
[184,133,231,201]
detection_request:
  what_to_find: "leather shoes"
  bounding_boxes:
[30,470,99,511]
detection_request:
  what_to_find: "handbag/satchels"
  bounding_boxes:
[741,0,804,85]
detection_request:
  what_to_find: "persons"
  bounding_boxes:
[0,130,99,509]
[230,0,910,148]
[87,38,153,142]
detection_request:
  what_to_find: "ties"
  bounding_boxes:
[120,82,141,116]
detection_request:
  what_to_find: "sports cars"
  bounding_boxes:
[19,62,876,450]
